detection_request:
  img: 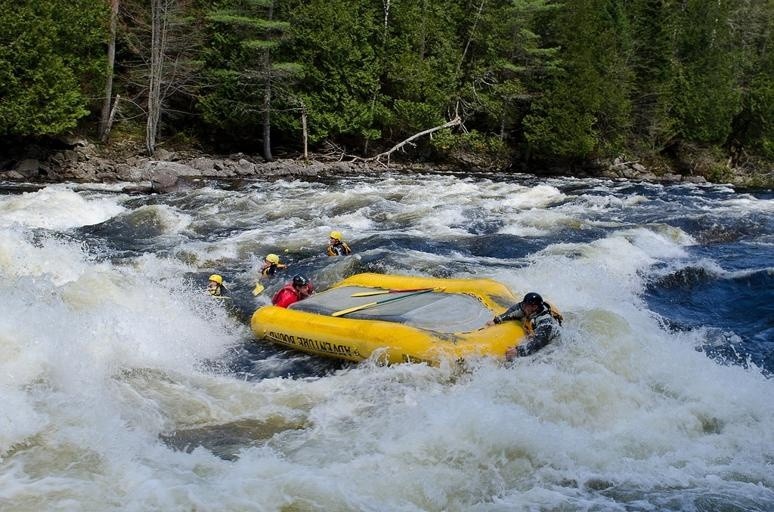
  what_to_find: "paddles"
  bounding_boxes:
[332,291,433,317]
[351,287,446,297]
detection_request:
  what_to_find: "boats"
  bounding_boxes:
[251,272,562,374]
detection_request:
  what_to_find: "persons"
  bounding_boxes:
[206,274,231,299]
[273,273,314,308]
[489,291,563,358]
[318,231,350,259]
[260,253,284,278]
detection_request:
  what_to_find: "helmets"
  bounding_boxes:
[208,274,224,284]
[522,292,543,305]
[329,230,343,242]
[266,254,279,265]
[292,273,309,287]
